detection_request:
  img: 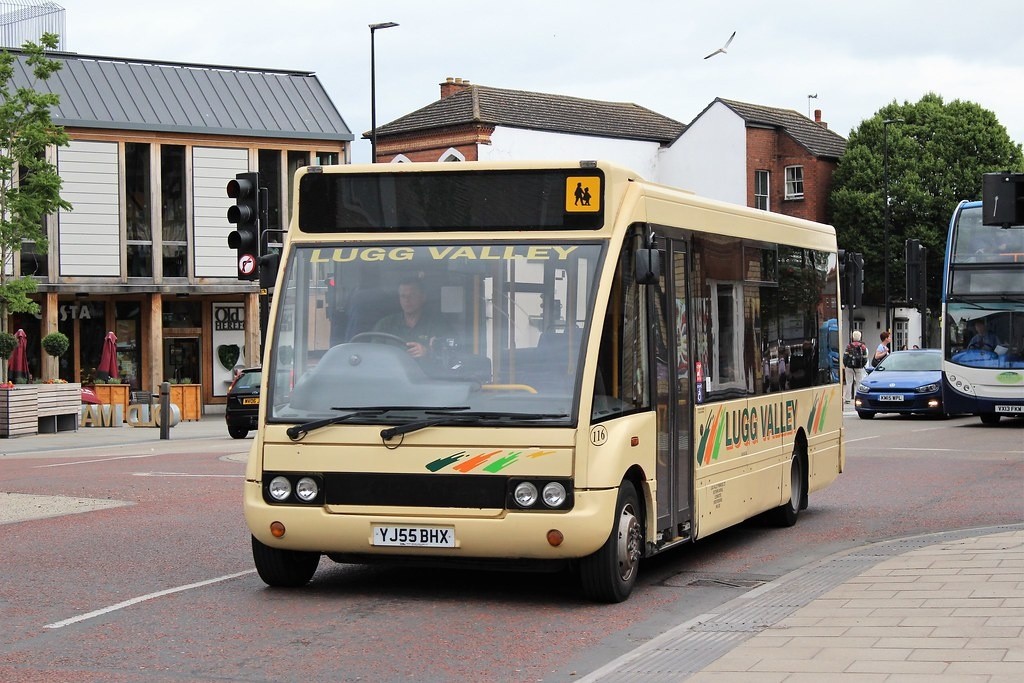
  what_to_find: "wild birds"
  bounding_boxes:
[703,30,737,59]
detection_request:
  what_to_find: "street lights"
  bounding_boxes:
[882,118,906,353]
[369,21,398,163]
[838,250,846,279]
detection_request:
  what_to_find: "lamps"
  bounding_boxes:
[176,293,189,299]
[75,293,90,298]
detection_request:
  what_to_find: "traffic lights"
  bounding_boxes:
[227,173,260,281]
[325,274,335,318]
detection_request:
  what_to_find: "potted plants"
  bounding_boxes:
[157,378,202,421]
[86,378,130,422]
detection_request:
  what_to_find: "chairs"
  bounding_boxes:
[536,323,580,367]
[131,390,154,421]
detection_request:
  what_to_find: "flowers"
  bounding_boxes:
[15,376,68,384]
[0,380,17,388]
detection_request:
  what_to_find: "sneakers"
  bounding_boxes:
[846,400,850,403]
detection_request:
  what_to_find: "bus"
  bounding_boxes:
[820,319,838,383]
[243,159,844,602]
[938,200,1024,426]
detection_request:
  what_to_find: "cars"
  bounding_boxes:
[854,348,949,418]
[226,366,296,440]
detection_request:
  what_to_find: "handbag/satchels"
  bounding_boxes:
[871,347,888,367]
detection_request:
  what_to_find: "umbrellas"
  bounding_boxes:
[8,329,30,384]
[97,331,118,384]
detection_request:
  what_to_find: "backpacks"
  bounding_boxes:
[847,342,865,368]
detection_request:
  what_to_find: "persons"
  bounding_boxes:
[860,341,870,372]
[967,322,1001,351]
[874,331,891,365]
[842,331,868,404]
[913,345,919,349]
[900,345,907,351]
[372,276,462,379]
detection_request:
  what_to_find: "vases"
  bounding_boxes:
[0,386,40,439]
[14,382,82,434]
